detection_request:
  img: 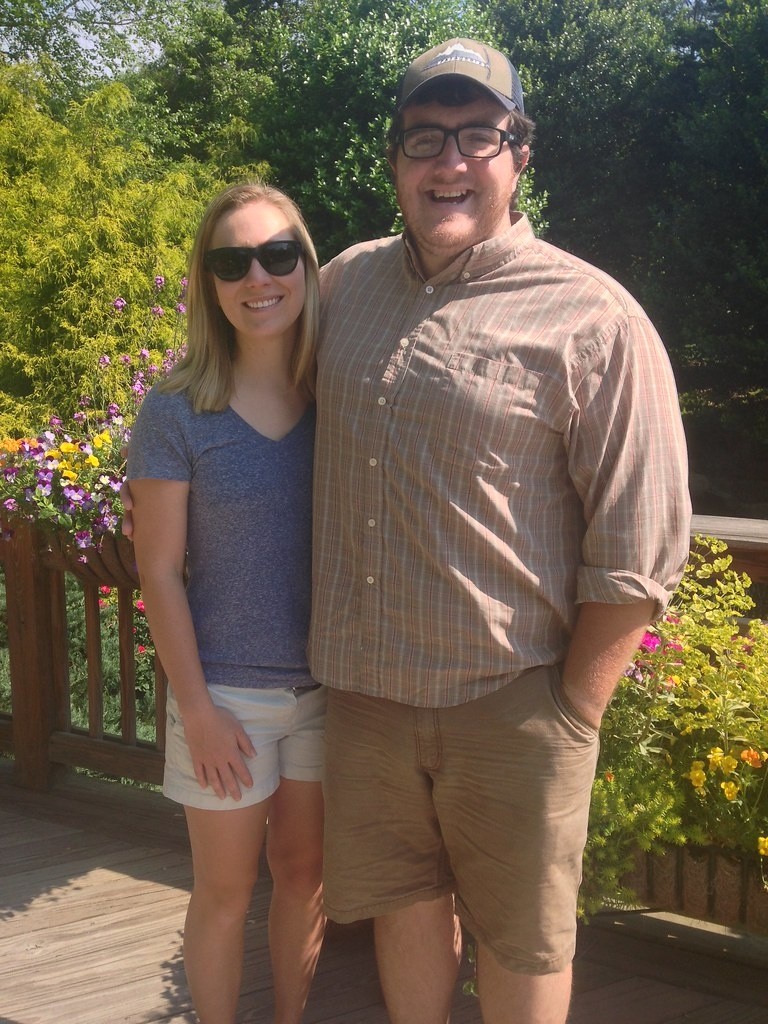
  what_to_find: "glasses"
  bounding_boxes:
[395,125,518,159]
[203,240,302,281]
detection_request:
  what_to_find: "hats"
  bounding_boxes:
[397,36,524,116]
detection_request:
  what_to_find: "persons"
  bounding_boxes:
[127,181,330,1024]
[121,38,691,1024]
